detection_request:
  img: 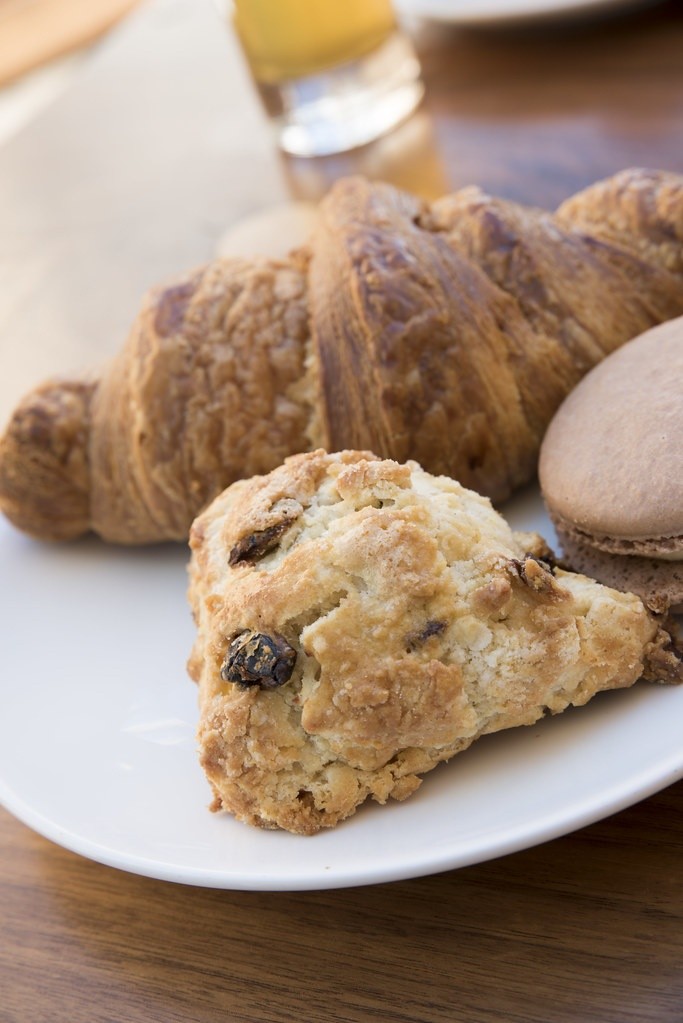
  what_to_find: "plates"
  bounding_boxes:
[0,8,683,893]
[416,1,627,29]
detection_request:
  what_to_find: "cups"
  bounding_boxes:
[220,1,426,160]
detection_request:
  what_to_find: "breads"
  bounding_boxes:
[0,165,683,832]
